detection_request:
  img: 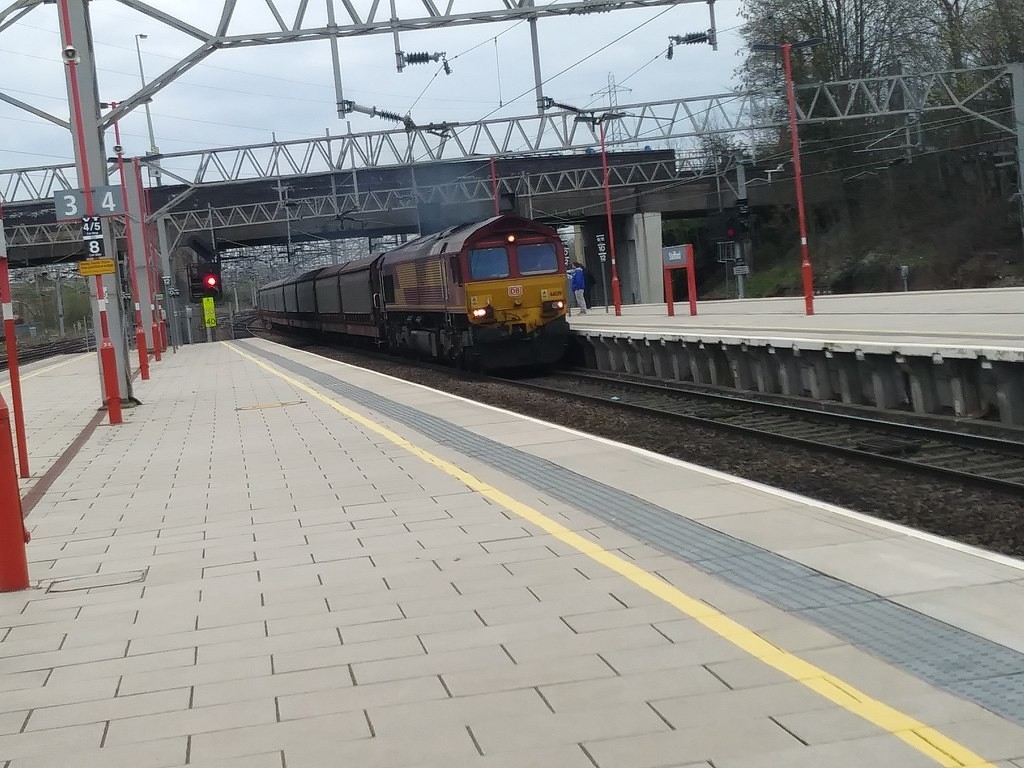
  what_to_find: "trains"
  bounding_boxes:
[255,215,574,373]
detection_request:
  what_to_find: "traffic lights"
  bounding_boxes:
[721,208,739,241]
[201,263,223,299]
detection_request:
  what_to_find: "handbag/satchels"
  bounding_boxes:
[585,269,596,285]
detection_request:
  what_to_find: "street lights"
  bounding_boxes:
[749,36,827,316]
[107,154,166,361]
[100,98,154,381]
[472,150,514,217]
[135,33,163,187]
[572,111,623,316]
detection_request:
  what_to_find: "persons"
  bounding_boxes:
[570,262,592,316]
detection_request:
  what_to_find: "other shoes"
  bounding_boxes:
[587,305,592,309]
[577,310,587,316]
